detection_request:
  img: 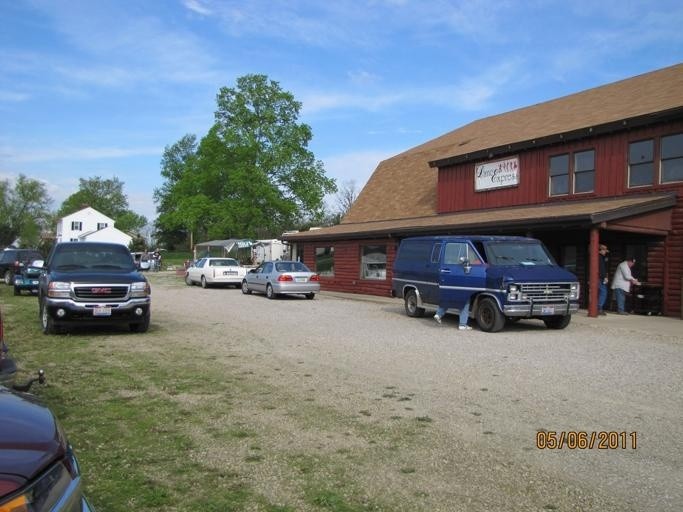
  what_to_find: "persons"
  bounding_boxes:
[585,243,611,317]
[609,255,642,317]
[183,258,190,274]
[12,254,23,296]
[153,251,163,271]
[139,249,150,275]
[433,243,481,330]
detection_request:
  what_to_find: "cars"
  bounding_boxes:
[0,242,161,297]
[183,257,245,288]
[0,385,93,511]
[240,261,321,299]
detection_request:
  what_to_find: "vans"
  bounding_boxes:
[392,235,581,332]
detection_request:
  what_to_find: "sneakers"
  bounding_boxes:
[458,325,471,330]
[433,315,441,325]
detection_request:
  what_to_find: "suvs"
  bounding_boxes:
[37,241,152,335]
